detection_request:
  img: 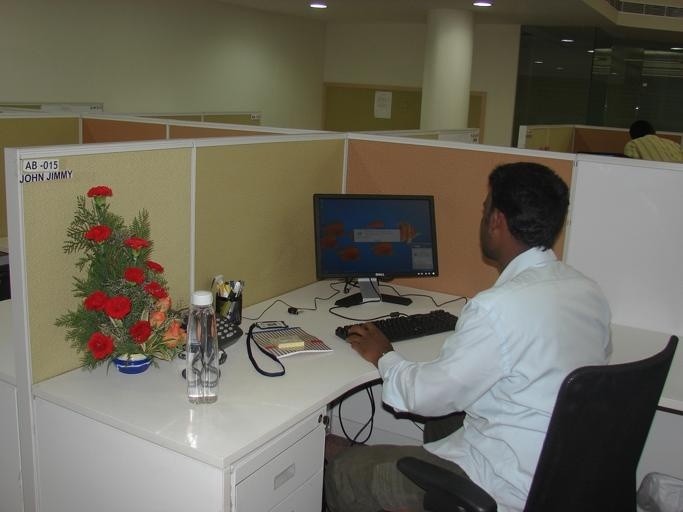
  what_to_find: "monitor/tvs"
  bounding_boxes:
[313,194,439,307]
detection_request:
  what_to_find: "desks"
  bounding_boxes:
[33,270,683,512]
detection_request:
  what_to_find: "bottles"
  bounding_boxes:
[186,290,218,403]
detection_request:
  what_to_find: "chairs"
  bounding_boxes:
[396,335,679,510]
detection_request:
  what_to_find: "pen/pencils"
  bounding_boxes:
[217,278,244,324]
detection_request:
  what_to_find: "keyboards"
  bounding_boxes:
[335,309,458,344]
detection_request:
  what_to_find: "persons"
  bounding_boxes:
[326,162,613,511]
[624,119,683,163]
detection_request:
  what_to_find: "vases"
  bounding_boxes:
[113,353,153,375]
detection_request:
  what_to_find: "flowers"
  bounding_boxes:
[61,184,191,375]
